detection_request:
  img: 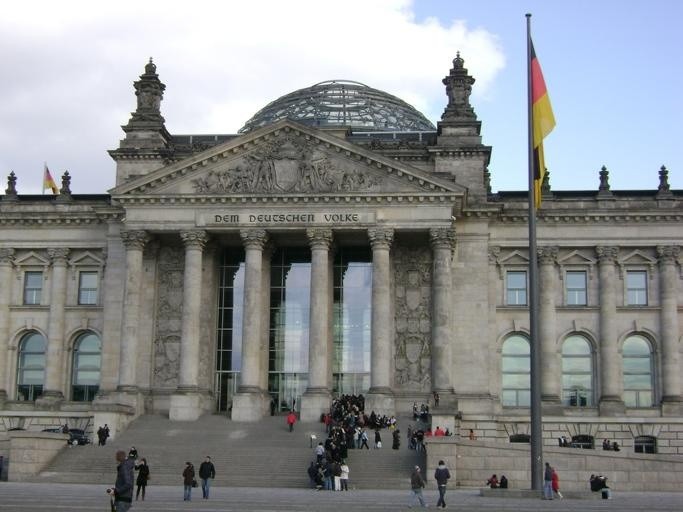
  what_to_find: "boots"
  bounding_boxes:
[556,492,563,502]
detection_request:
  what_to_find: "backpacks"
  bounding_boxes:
[333,462,341,475]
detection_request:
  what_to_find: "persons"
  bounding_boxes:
[487,437,620,499]
[97,424,150,512]
[183,462,194,500]
[59,424,68,433]
[288,412,296,432]
[271,400,276,416]
[408,466,428,509]
[434,462,451,509]
[199,456,215,499]
[308,394,475,491]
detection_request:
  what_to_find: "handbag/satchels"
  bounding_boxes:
[193,477,198,487]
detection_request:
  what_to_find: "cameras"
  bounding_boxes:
[107,488,115,493]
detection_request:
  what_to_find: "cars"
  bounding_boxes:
[40,429,90,446]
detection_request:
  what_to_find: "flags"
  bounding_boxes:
[531,37,555,210]
[44,166,58,194]
